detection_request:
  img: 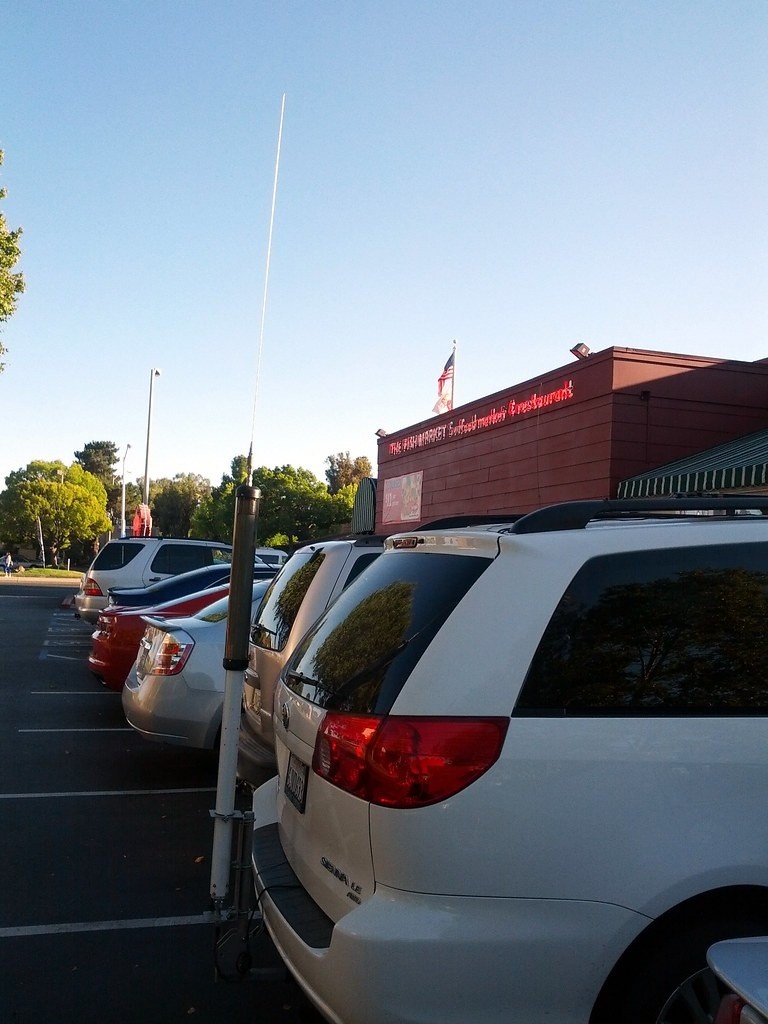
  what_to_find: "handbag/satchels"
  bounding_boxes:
[10,561,13,567]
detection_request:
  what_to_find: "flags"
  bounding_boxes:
[438,352,454,395]
[432,385,450,413]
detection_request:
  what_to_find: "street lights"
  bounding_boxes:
[120,443,132,538]
[134,368,160,537]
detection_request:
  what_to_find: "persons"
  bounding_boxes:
[4,552,12,578]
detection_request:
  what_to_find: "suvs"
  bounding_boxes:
[73,537,266,624]
[237,532,393,799]
[237,492,768,1024]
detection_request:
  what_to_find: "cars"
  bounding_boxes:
[106,563,281,608]
[86,578,265,697]
[0,554,44,570]
[117,575,278,748]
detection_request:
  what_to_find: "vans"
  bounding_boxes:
[250,546,288,566]
